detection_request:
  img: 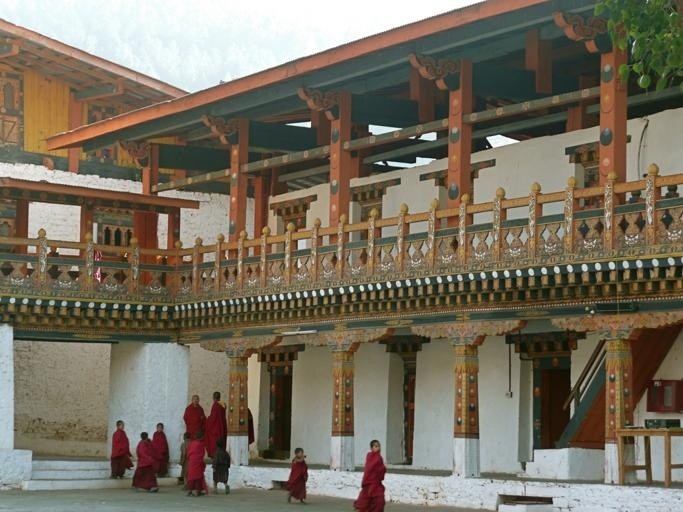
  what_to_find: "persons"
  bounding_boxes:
[247,407,254,451]
[177,431,192,490]
[183,394,206,442]
[199,391,227,464]
[352,439,387,511]
[210,438,230,495]
[286,447,308,504]
[151,422,170,477]
[131,431,159,492]
[109,420,133,479]
[183,431,206,497]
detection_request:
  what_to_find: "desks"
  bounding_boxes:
[611,426,683,487]
